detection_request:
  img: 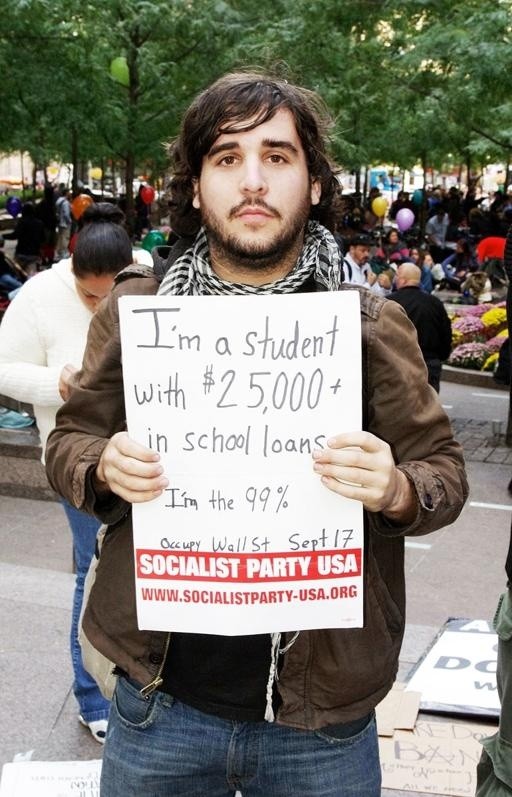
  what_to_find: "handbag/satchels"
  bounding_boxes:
[76,519,118,699]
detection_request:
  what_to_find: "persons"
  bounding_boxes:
[0,199,153,743]
[311,167,511,396]
[43,60,469,797]
[1,167,177,430]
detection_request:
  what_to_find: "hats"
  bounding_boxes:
[350,235,375,246]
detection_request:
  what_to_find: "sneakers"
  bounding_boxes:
[78,714,108,744]
